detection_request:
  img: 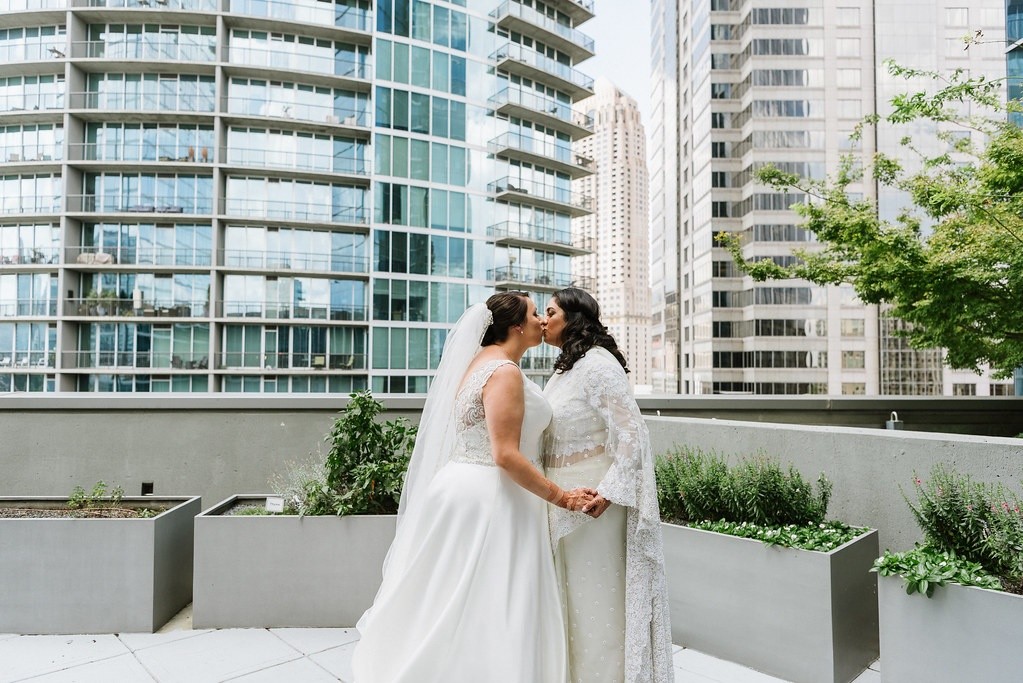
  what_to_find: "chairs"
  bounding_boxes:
[0,356,10,367]
[36,357,44,366]
[17,357,28,366]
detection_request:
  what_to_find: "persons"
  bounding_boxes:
[542,289,674,683]
[357,293,598,683]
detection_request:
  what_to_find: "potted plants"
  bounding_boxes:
[86,289,98,315]
[96,288,108,315]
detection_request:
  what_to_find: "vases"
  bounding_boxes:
[79,304,86,316]
